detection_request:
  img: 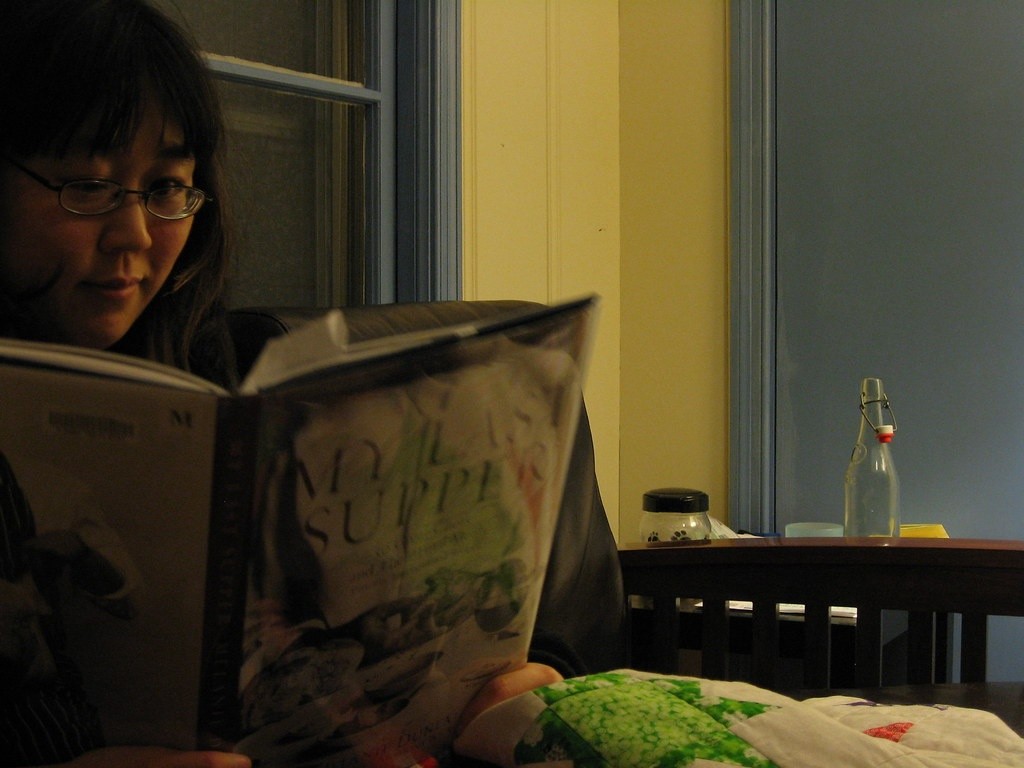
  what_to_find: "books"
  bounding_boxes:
[0,295,597,768]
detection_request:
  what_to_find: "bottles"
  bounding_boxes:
[639,487,712,605]
[844,377,900,536]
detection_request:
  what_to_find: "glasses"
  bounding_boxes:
[0,152,214,220]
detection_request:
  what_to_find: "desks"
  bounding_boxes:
[629,603,857,688]
[775,681,1024,738]
[624,537,1024,694]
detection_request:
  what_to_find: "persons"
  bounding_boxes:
[1,0,590,768]
